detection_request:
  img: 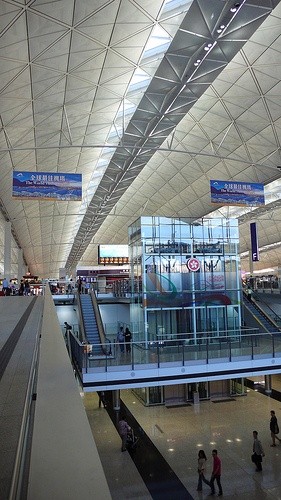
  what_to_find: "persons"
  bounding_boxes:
[124,328,132,353]
[196,450,210,492]
[246,274,279,289]
[98,391,106,408]
[210,449,223,496]
[252,431,265,472]
[118,415,132,451]
[64,322,70,337]
[117,327,126,353]
[1,275,142,296]
[246,287,254,300]
[270,410,281,446]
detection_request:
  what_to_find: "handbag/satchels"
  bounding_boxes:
[251,454,262,462]
